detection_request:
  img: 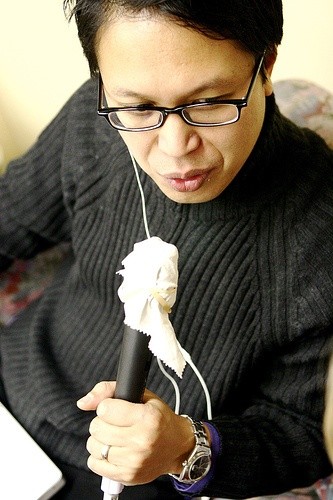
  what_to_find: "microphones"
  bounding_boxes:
[100,237,179,495]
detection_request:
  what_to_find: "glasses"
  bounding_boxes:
[95,46,268,133]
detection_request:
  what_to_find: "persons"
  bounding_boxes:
[321,354,333,461]
[1,0,333,499]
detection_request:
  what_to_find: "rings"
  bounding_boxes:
[102,444,111,460]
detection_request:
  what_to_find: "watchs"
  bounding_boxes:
[169,415,212,485]
